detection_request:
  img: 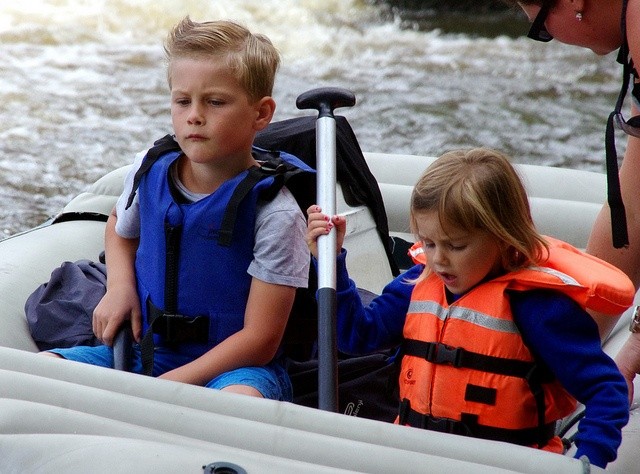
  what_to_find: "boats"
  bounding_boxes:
[0,151,639,474]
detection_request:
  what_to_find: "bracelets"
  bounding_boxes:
[629,305,640,333]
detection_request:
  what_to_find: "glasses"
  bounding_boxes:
[527,0,555,42]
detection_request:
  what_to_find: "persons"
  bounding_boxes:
[506,0,640,410]
[36,16,311,402]
[305,146,630,470]
